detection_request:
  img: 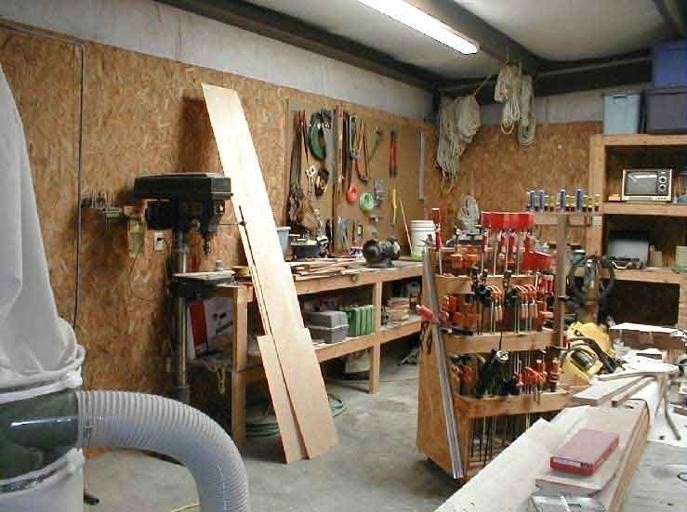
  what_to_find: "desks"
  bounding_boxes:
[168,256,426,453]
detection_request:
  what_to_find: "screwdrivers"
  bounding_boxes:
[529,189,601,227]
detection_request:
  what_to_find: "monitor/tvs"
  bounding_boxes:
[621,168,672,201]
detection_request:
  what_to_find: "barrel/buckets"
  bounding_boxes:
[409,219,437,260]
[277,225,292,260]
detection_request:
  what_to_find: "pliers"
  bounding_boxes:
[389,130,398,179]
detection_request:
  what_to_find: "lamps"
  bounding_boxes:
[357,1,480,56]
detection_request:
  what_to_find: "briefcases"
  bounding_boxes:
[601,258,641,270]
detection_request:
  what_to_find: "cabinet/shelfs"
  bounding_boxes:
[415,245,570,481]
[583,133,687,331]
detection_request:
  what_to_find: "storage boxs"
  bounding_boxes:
[600,42,687,134]
